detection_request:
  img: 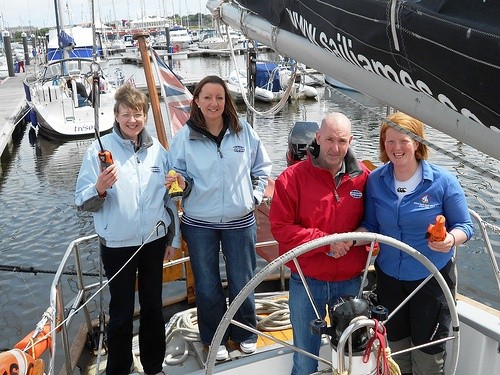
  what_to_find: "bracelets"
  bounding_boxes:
[352,238,357,246]
[449,233,456,248]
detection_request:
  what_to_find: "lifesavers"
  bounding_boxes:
[13,315,62,359]
[0,349,44,375]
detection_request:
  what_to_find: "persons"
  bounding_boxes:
[270,113,372,375]
[328,112,474,375]
[14,47,38,73]
[164,75,271,360]
[66,75,105,105]
[75,85,182,375]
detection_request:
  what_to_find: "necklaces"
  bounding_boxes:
[394,165,419,182]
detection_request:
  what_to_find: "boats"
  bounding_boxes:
[0,0,264,80]
[23,57,115,135]
[228,39,319,100]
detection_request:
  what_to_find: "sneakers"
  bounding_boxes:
[240,341,256,353]
[215,345,228,360]
